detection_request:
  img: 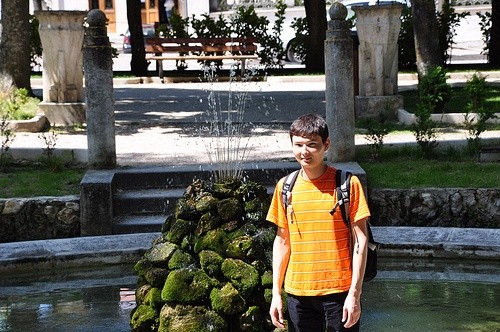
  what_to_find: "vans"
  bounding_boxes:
[120,25,159,52]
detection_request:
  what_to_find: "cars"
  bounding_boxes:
[286,0,423,66]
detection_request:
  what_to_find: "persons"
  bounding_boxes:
[265,114,371,332]
[164,0,175,24]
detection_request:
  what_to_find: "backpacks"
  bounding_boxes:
[282,169,379,283]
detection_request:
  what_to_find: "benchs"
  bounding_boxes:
[146,37,257,81]
